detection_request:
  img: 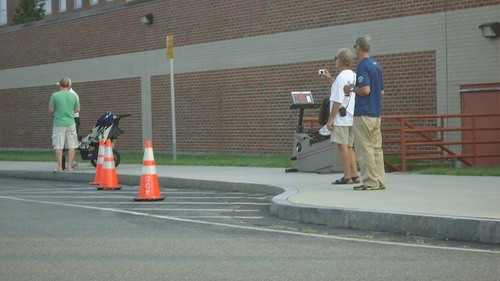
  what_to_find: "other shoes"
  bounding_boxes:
[353,182,385,190]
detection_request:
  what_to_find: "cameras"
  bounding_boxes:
[319,70,325,75]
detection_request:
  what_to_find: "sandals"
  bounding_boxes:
[332,175,360,184]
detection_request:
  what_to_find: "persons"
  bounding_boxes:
[343,36,386,191]
[48,77,80,173]
[324,47,361,185]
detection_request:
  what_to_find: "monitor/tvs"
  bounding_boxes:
[291,92,314,104]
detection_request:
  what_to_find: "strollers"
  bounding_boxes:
[77,112,132,169]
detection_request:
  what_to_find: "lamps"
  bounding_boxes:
[479,22,500,38]
[139,14,154,25]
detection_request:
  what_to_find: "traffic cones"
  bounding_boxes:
[131,140,167,201]
[97,139,123,190]
[88,137,106,185]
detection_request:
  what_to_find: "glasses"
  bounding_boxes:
[353,44,357,48]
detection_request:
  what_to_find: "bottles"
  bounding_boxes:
[344,80,351,96]
